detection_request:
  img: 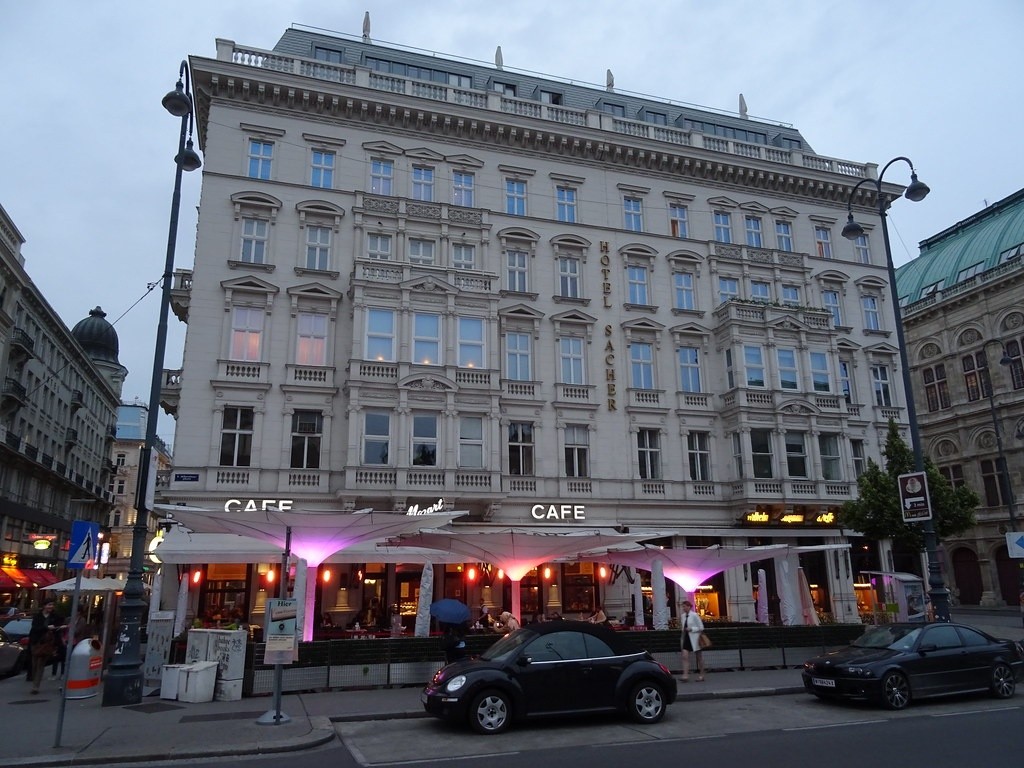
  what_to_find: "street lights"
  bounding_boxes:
[982,339,1018,533]
[100,60,202,707]
[842,158,949,621]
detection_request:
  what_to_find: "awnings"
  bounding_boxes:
[0,566,62,589]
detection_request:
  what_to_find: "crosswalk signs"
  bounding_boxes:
[66,519,100,569]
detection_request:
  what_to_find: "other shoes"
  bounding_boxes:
[31,686,40,695]
[59,674,64,680]
[678,677,689,681]
[48,675,56,680]
[696,678,704,682]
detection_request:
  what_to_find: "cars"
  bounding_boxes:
[802,620,1023,710]
[0,627,25,678]
[420,619,677,734]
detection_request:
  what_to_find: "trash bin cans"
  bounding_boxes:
[65,638,102,699]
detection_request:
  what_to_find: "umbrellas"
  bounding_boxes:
[39,576,153,596]
[429,599,470,621]
[798,566,820,628]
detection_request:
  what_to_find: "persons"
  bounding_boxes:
[205,602,244,625]
[480,598,607,629]
[51,618,66,681]
[321,597,393,636]
[674,600,706,682]
[66,603,86,657]
[439,620,466,665]
[25,598,54,695]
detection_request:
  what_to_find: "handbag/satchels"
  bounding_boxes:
[699,631,713,648]
